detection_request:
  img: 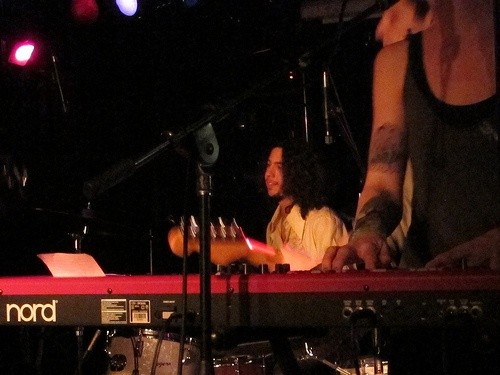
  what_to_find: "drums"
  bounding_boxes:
[104,329,204,374]
[208,352,272,375]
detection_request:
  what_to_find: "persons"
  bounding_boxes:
[311,2,499,375]
[253,133,358,276]
[358,149,413,257]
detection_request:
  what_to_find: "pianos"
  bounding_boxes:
[0,266,500,331]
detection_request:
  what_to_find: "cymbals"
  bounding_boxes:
[42,222,117,239]
[35,206,121,226]
